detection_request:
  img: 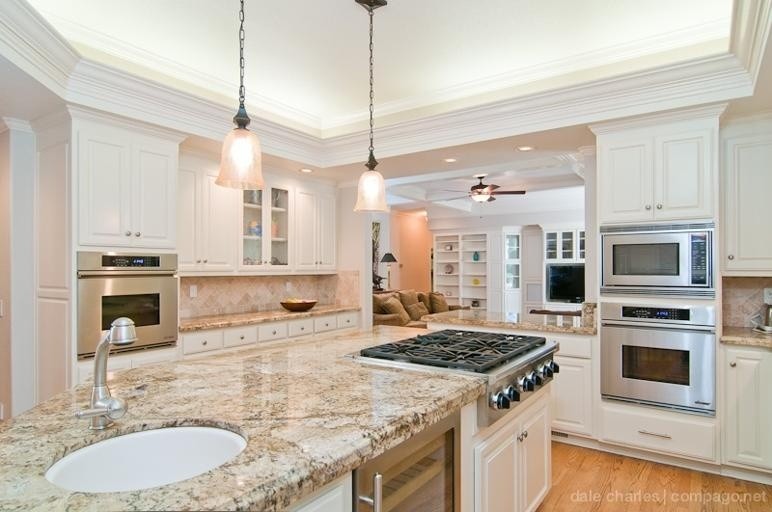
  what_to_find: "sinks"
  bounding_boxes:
[44,418,248,493]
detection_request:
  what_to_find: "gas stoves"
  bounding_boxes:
[361,328,562,432]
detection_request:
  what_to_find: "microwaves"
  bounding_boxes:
[600,233,710,289]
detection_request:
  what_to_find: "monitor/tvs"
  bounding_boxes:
[546,263,585,303]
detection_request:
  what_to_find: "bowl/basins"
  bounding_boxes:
[281,299,319,313]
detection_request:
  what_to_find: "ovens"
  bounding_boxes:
[598,319,716,420]
[78,271,180,359]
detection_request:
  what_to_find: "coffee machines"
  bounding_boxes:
[759,288,771,333]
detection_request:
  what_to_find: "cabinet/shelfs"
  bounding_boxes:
[33,110,187,248]
[718,116,772,278]
[717,341,772,486]
[463,394,554,511]
[596,403,717,478]
[550,333,596,443]
[544,229,585,262]
[179,311,360,355]
[429,231,488,318]
[586,102,728,231]
[242,187,288,276]
[288,173,339,275]
[175,150,242,276]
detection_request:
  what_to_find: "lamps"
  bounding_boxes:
[214,1,265,193]
[352,1,393,215]
[380,252,397,286]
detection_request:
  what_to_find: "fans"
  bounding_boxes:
[427,177,527,206]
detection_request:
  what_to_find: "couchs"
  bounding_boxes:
[372,290,471,329]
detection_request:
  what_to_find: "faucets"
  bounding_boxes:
[89,317,138,433]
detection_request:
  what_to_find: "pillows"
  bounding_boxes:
[381,290,450,324]
